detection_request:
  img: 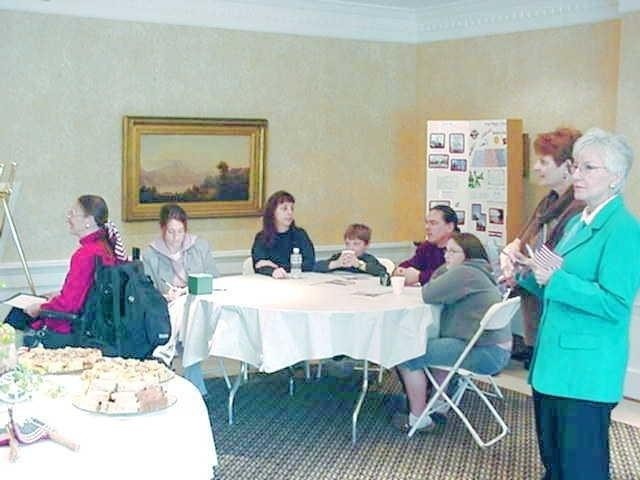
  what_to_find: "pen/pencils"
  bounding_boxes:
[161,278,175,292]
[213,289,226,291]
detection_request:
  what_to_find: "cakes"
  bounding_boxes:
[71,360,171,416]
[78,357,177,385]
[17,344,103,376]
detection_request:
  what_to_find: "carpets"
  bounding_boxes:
[177,364,638,480]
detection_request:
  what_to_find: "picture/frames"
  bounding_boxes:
[123,115,268,221]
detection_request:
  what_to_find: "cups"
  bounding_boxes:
[391,277,405,296]
[342,251,355,268]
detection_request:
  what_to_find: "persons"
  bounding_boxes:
[394,234,513,430]
[526,129,640,480]
[392,205,460,286]
[140,204,221,403]
[514,130,587,375]
[313,224,386,276]
[251,191,316,278]
[23,195,127,349]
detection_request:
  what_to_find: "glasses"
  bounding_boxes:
[567,162,606,176]
[443,248,463,256]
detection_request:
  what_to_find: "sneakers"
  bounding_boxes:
[391,414,447,434]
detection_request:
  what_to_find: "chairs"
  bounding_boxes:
[315,257,395,382]
[24,248,170,359]
[407,295,521,449]
[240,256,313,395]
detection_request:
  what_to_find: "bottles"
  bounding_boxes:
[290,248,302,279]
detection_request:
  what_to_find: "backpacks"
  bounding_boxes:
[118,259,171,357]
